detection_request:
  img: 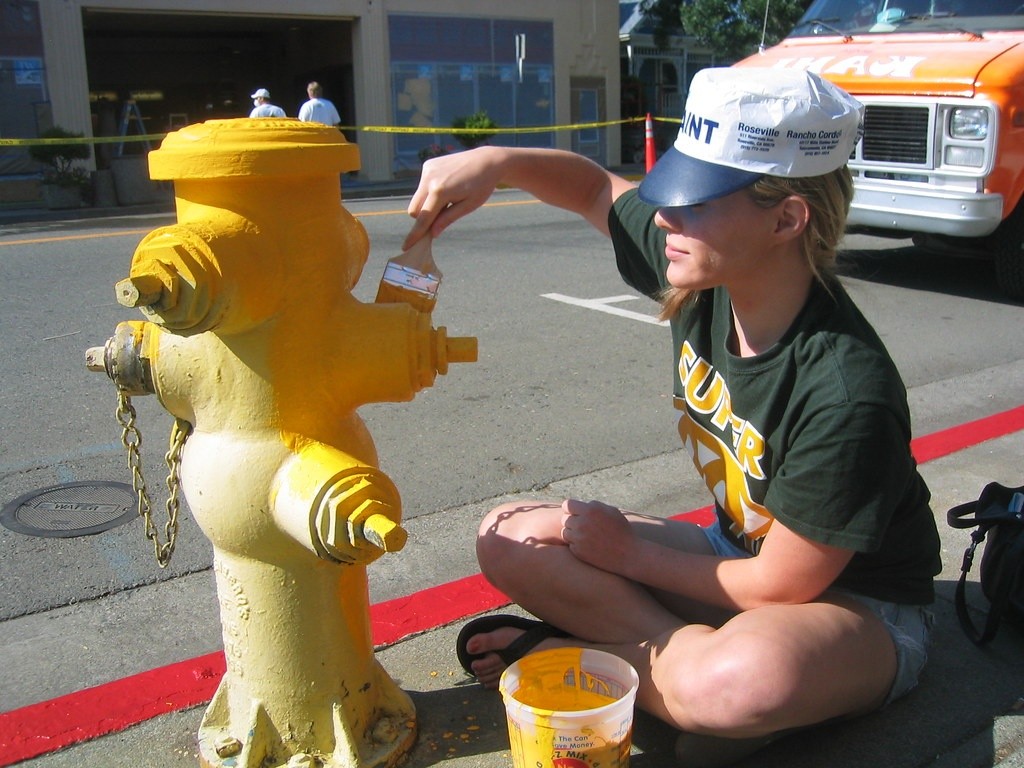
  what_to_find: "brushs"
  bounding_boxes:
[374,224,443,313]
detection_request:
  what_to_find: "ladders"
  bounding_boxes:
[119,97,153,154]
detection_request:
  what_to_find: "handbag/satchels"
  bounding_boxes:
[946,481,1024,647]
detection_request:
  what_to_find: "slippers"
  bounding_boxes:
[455,616,573,691]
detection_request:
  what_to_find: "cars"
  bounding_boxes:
[725,0,1024,258]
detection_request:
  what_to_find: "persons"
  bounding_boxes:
[298,82,340,126]
[248,88,287,118]
[402,65,942,740]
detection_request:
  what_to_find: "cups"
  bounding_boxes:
[499,647,640,768]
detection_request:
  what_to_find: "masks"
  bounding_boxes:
[254,100,260,107]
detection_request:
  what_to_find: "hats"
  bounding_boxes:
[250,89,271,99]
[636,56,861,205]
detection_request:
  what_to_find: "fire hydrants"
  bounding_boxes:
[84,117,481,768]
